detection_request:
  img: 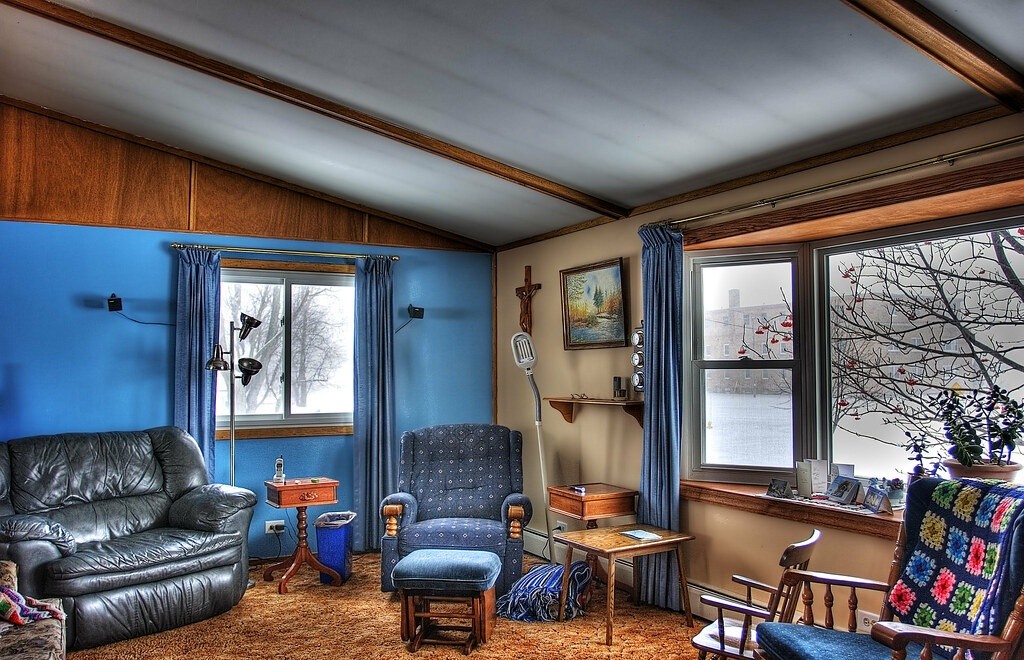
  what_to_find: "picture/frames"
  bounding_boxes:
[559,256,630,352]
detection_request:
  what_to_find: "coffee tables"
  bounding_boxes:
[262,477,343,594]
[546,483,639,590]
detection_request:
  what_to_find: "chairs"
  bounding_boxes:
[752,466,1024,660]
[690,529,821,660]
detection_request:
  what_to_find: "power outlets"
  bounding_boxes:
[556,520,567,533]
[856,609,880,633]
[264,519,285,534]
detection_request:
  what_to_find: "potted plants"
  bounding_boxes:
[929,385,1024,481]
[886,478,904,499]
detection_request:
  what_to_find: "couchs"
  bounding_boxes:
[0,424,258,660]
[379,423,534,601]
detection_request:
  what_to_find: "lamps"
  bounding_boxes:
[205,312,262,486]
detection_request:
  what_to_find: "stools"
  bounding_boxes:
[390,548,502,655]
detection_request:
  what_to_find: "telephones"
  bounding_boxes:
[272,454,286,483]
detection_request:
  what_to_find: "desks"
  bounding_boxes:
[552,523,696,646]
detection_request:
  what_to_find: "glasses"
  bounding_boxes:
[571,393,588,399]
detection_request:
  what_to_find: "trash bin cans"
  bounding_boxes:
[311,510,357,586]
